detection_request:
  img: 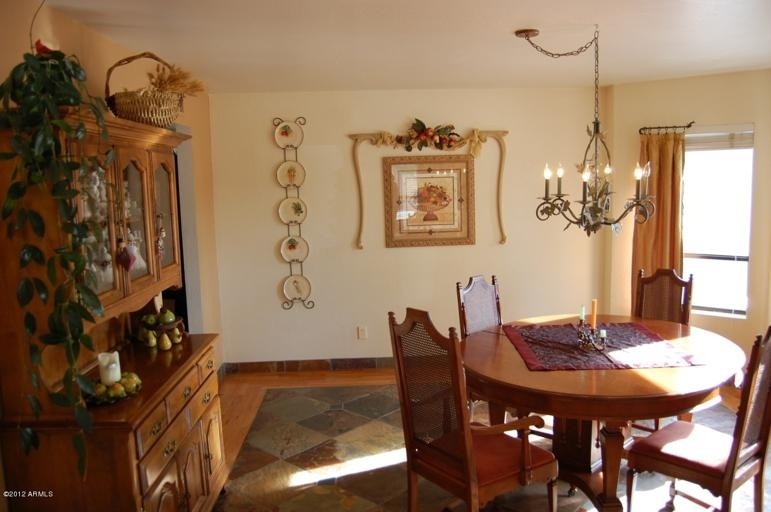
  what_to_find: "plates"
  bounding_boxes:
[276,160,307,190]
[280,235,310,265]
[275,120,305,151]
[278,197,308,227]
[94,376,142,404]
[283,274,313,304]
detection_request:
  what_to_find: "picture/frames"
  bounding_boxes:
[382,156,476,249]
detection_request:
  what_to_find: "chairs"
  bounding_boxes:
[631,268,693,326]
[455,275,503,340]
[385,306,561,512]
[626,322,771,512]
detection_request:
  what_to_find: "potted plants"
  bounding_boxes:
[1,44,124,482]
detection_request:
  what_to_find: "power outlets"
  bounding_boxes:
[356,326,368,341]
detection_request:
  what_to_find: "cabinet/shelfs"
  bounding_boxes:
[0,107,231,512]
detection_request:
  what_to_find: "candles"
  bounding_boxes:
[599,328,608,338]
[591,298,598,332]
[579,304,587,322]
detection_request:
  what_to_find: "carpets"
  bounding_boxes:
[212,383,771,512]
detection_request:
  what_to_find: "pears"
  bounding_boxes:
[158,332,174,351]
[170,327,182,343]
[159,308,176,325]
[146,314,156,324]
[145,329,157,347]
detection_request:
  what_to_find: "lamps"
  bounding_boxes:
[513,24,660,238]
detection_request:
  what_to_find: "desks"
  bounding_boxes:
[460,312,749,512]
